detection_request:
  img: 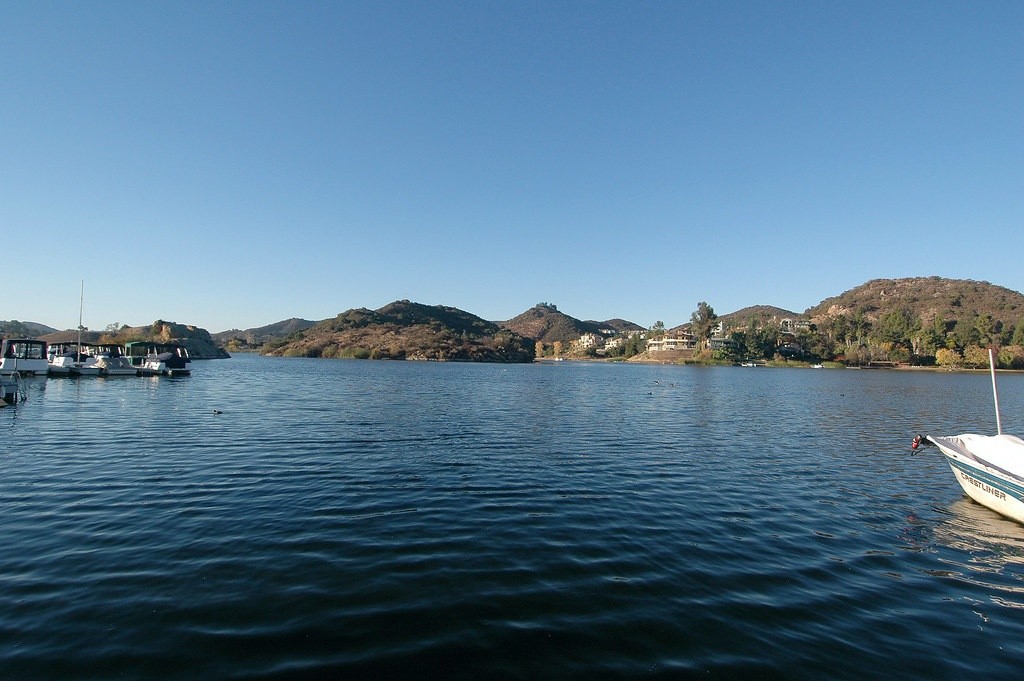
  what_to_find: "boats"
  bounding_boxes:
[741,362,757,368]
[0,279,195,407]
[911,349,1024,524]
[810,363,825,369]
